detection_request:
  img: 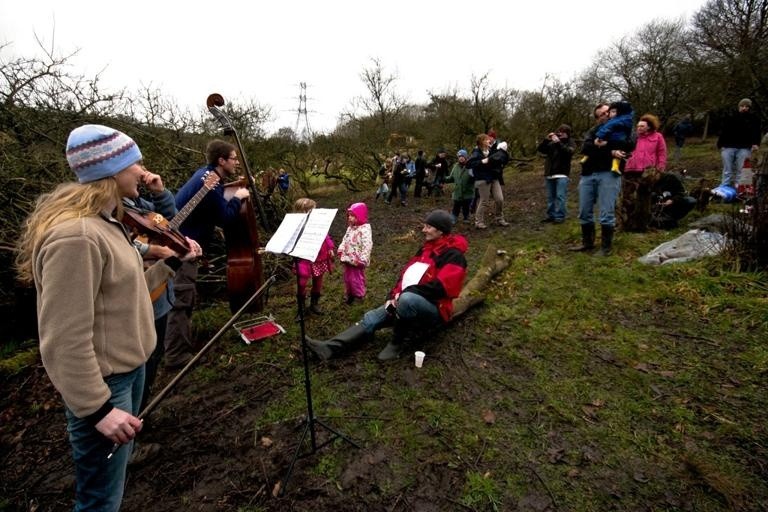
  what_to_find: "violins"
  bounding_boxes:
[114,207,191,254]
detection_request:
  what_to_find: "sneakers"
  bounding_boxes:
[475,222,486,229]
[594,246,612,257]
[541,218,562,223]
[497,220,509,226]
[569,243,593,251]
[377,339,405,361]
[305,335,331,360]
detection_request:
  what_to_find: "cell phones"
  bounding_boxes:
[385,303,397,315]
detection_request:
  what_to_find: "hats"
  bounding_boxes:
[424,210,452,234]
[67,124,142,184]
[737,98,752,106]
[457,150,467,157]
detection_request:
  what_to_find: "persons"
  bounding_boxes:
[162,139,255,372]
[376,128,513,228]
[303,209,467,359]
[264,169,290,200]
[716,95,758,193]
[291,198,336,321]
[124,169,200,466]
[623,113,667,234]
[674,129,684,158]
[576,102,632,174]
[338,203,376,302]
[538,122,572,223]
[568,102,635,255]
[9,124,162,511]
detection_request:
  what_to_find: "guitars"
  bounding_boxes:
[129,170,220,302]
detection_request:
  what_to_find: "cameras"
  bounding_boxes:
[549,133,561,138]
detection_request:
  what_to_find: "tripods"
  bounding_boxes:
[278,258,361,495]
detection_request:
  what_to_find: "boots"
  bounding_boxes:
[577,156,588,163]
[296,296,305,321]
[611,158,623,175]
[310,293,322,314]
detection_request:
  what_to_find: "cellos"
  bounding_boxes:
[207,93,264,316]
